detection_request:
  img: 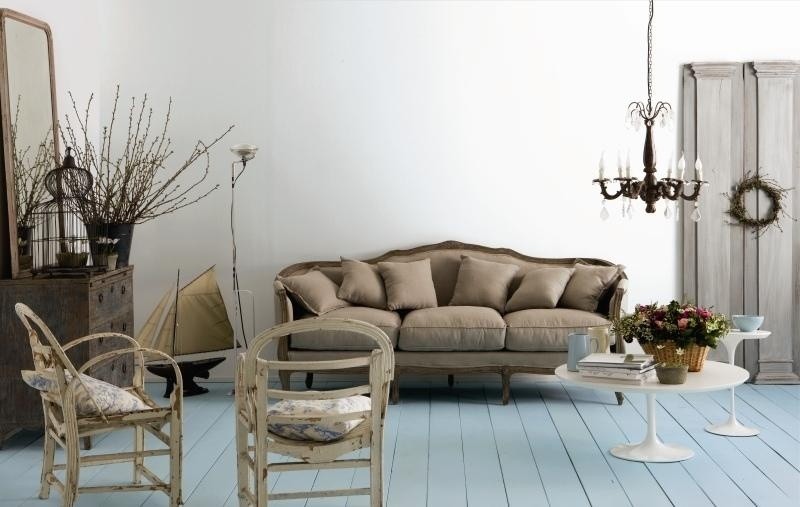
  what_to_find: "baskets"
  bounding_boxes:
[637,332,714,372]
[55,251,88,267]
[91,253,110,265]
[17,226,33,268]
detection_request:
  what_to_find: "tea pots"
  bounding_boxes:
[587,325,611,353]
[567,331,600,370]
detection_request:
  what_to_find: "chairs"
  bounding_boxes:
[11,293,190,507]
[227,315,404,507]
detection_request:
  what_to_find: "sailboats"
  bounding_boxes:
[132,262,243,378]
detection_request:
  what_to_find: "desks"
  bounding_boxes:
[706,328,773,441]
[553,356,750,466]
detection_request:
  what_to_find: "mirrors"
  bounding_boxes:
[1,2,68,274]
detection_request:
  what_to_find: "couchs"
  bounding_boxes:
[269,235,629,407]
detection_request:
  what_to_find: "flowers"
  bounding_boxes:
[614,299,734,348]
[94,234,119,254]
[652,344,687,365]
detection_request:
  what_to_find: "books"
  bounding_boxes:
[575,352,662,384]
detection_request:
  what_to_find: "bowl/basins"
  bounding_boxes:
[732,314,765,332]
[655,360,689,384]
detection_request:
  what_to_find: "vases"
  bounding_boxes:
[82,219,138,265]
[94,253,117,270]
[655,363,690,384]
[17,226,36,268]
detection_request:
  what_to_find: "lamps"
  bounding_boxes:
[226,140,261,353]
[588,0,707,226]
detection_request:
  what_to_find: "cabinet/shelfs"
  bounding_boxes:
[674,52,798,392]
[1,257,140,454]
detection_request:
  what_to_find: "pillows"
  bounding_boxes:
[13,359,152,419]
[260,389,373,441]
